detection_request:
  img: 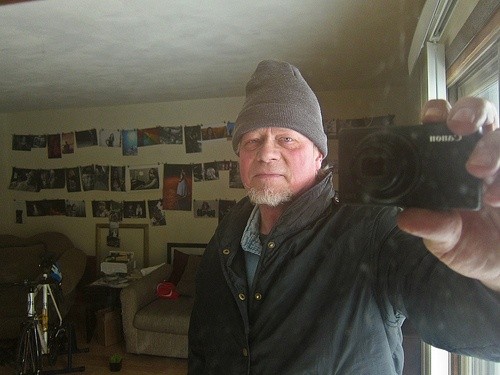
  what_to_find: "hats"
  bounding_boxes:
[231,60,329,158]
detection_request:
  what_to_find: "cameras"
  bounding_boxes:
[337,120,482,212]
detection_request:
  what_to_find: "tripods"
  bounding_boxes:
[34,284,62,355]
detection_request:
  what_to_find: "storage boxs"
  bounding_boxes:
[101,261,134,273]
[97,308,120,347]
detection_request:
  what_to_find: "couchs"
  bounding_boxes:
[120,263,200,359]
[0,233,87,340]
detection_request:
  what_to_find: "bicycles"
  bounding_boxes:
[3,262,90,374]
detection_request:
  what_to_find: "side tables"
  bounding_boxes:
[83,273,130,345]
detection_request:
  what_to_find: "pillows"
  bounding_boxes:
[169,249,187,286]
[177,256,201,296]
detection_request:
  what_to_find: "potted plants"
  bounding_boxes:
[107,352,123,372]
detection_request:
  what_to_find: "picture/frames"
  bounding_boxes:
[168,244,208,264]
[95,223,148,271]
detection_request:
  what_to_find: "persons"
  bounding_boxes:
[8,126,244,225]
[188,60,500,375]
